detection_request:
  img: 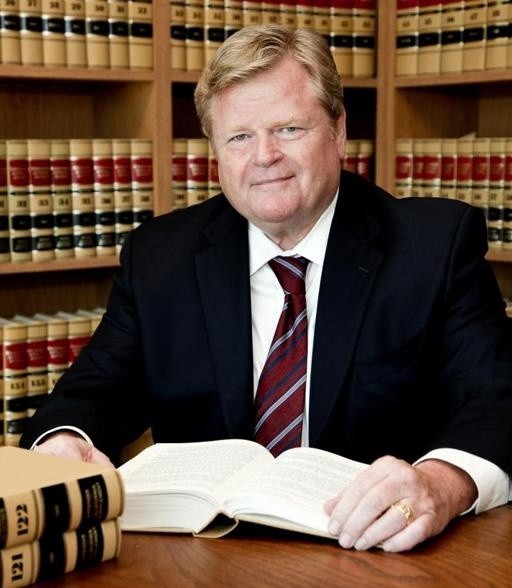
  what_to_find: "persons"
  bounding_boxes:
[17,23,512,553]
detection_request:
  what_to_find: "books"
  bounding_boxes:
[397,1,512,74]
[0,1,153,70]
[0,446,125,549]
[114,439,386,553]
[1,308,106,442]
[340,141,376,177]
[170,0,378,76]
[1,139,153,263]
[396,138,512,249]
[0,517,122,588]
[171,139,223,208]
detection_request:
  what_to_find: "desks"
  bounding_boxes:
[36,501,512,588]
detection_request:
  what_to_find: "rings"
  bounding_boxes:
[391,500,416,526]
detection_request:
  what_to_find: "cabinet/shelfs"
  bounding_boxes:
[386,1,512,263]
[2,0,385,274]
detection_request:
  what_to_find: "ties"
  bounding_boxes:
[253,254,309,460]
